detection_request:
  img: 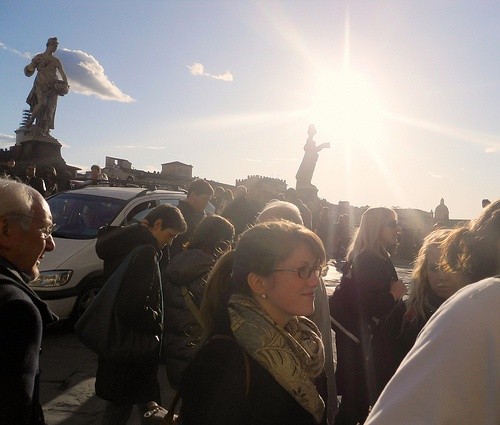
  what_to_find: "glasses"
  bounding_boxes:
[10,213,56,241]
[380,222,399,227]
[257,264,323,280]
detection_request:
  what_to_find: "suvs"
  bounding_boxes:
[28,178,189,327]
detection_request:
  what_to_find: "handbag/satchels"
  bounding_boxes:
[73,244,163,372]
[329,244,401,360]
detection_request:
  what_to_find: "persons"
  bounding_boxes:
[434,198,450,225]
[295,124,330,187]
[364,229,482,412]
[177,178,357,259]
[95,204,187,425]
[329,207,408,425]
[0,158,134,197]
[162,213,235,425]
[364,199,500,425]
[178,221,328,425]
[0,179,59,425]
[254,200,339,425]
[24,37,69,134]
